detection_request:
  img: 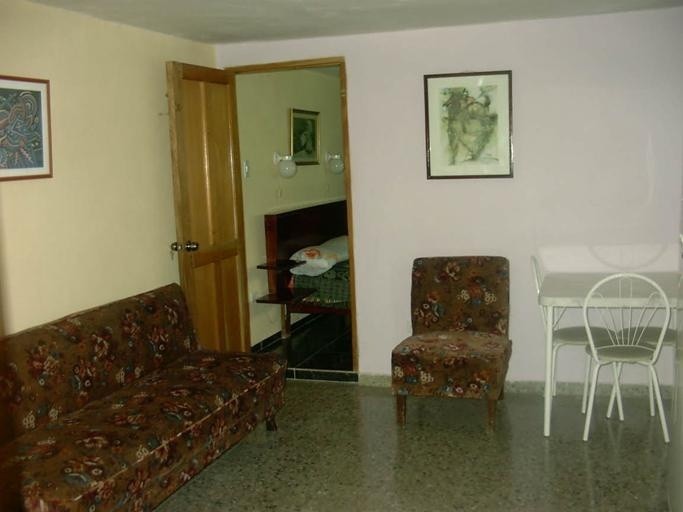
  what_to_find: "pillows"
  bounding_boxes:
[289,236,349,276]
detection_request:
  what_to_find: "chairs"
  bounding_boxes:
[530,256,682,442]
[391,255,513,436]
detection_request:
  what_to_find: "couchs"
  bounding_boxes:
[0,282,288,512]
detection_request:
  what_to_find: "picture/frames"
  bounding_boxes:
[423,70,514,180]
[290,106,321,165]
[0,74,52,181]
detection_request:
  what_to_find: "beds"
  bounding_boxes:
[256,197,351,340]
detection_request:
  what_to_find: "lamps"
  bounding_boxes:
[325,151,345,175]
[273,151,297,177]
